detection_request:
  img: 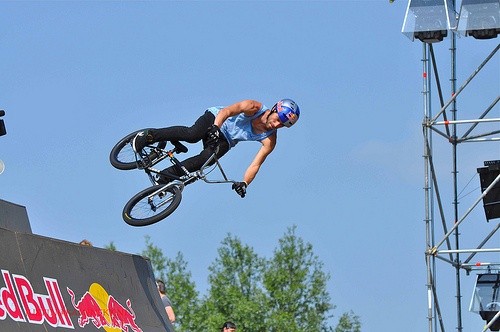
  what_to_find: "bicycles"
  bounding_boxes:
[109,128,247,227]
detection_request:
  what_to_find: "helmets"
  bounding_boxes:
[276,98,300,128]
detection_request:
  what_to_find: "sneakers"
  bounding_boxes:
[153,173,167,197]
[133,127,153,152]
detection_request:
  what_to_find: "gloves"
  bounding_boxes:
[205,125,222,148]
[232,181,247,197]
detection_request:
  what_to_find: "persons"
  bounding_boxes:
[133,98,300,195]
[221,321,235,332]
[156,280,175,322]
